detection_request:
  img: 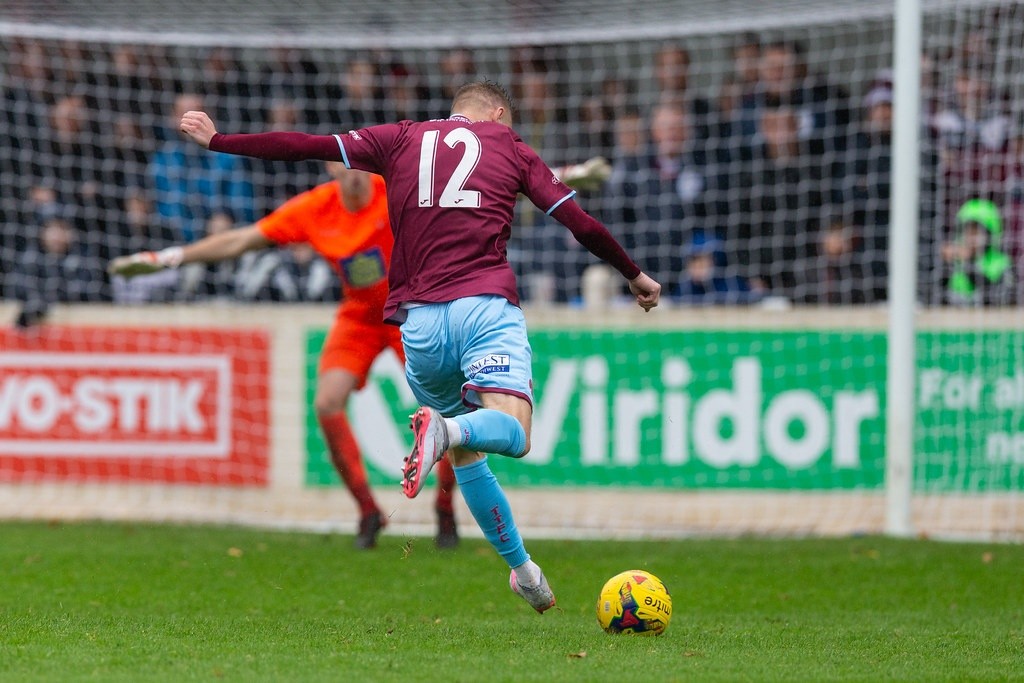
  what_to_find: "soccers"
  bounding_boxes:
[595,567,675,637]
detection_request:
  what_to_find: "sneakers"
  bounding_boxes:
[510,569,556,614]
[400,406,450,498]
[357,510,387,550]
[434,500,459,548]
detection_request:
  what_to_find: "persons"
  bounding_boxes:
[180,80,661,614]
[109,161,458,552]
[0,23,1024,323]
